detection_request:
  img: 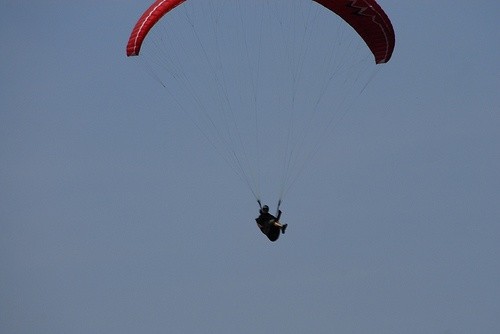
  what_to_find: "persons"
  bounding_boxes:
[259,204,288,241]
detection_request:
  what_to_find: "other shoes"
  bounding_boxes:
[282,223,287,234]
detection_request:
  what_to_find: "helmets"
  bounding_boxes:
[262,205,269,213]
